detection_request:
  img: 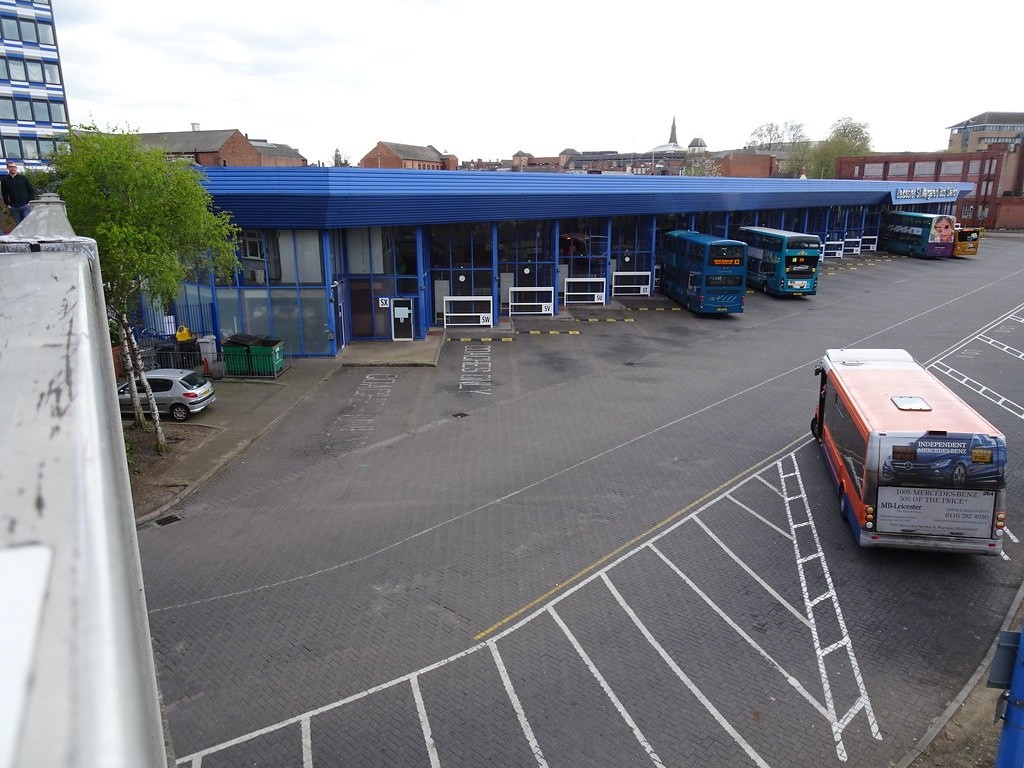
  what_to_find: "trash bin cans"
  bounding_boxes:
[222,332,284,376]
[154,334,217,370]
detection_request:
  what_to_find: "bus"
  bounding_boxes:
[737,225,822,297]
[952,227,980,255]
[659,229,749,316]
[811,346,1008,564]
[877,210,957,259]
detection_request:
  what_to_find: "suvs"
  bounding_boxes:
[882,432,1007,490]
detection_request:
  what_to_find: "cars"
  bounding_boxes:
[118,368,216,422]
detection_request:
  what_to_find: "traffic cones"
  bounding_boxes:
[201,358,212,377]
